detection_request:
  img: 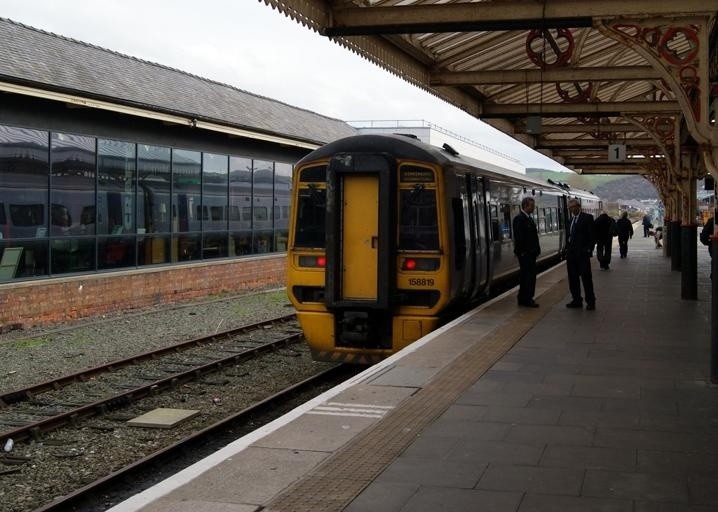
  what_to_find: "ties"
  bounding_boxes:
[570,217,577,239]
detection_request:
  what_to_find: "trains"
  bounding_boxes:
[0,174,292,247]
[284,132,603,365]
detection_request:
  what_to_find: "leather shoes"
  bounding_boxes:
[586,304,595,310]
[517,299,539,308]
[566,303,583,308]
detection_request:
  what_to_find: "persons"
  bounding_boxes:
[560,198,599,311]
[511,196,541,309]
[593,208,617,270]
[615,211,634,259]
[648,230,663,249]
[641,213,651,238]
[700,216,717,284]
